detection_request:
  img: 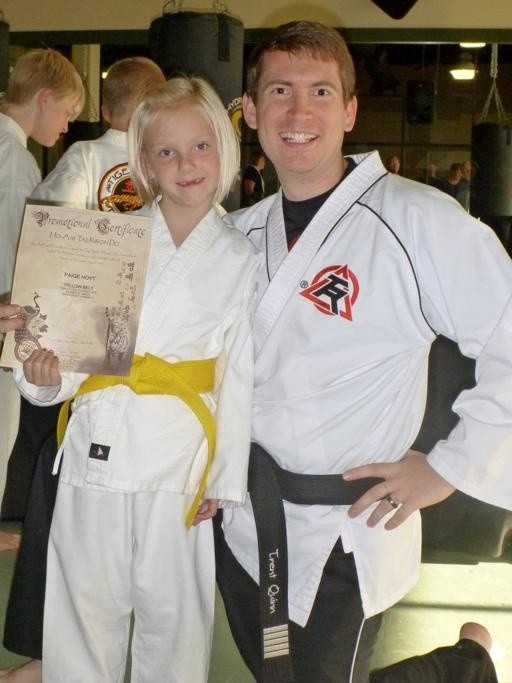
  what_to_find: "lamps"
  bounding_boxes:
[447,51,482,85]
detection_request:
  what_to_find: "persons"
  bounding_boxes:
[1,22,512,682]
[2,57,165,682]
[2,48,85,495]
[384,155,401,178]
[243,148,266,205]
[435,162,463,196]
[23,76,259,683]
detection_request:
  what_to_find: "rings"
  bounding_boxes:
[386,494,401,509]
